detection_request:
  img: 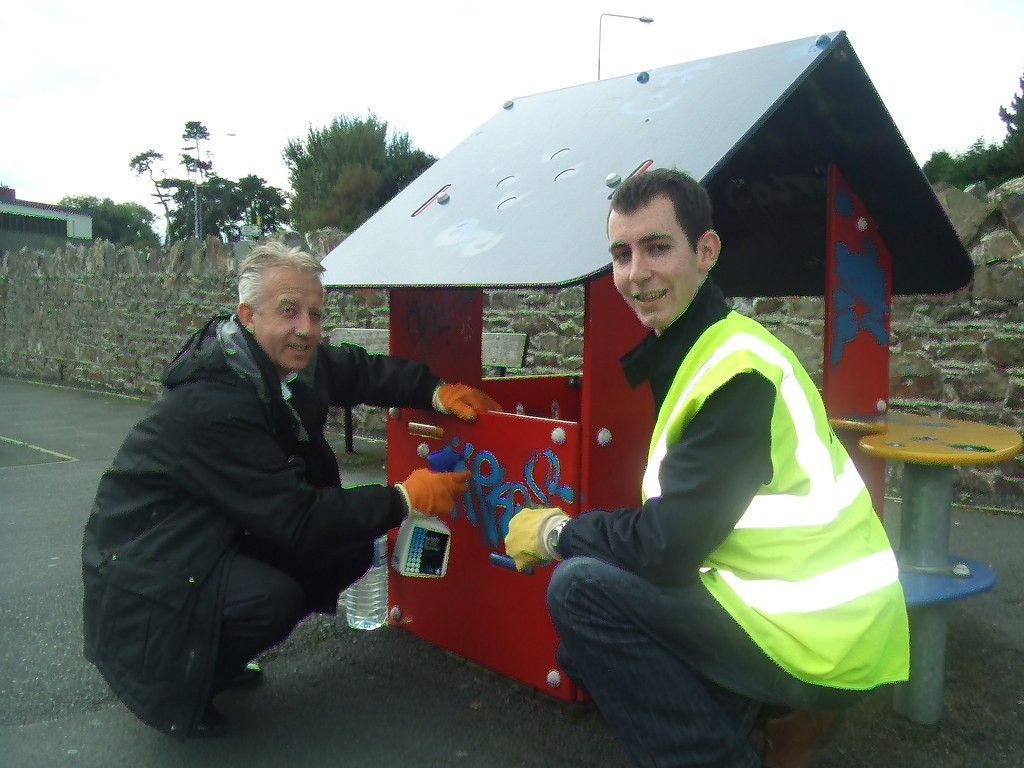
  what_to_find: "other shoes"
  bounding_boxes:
[196,703,228,737]
[222,661,262,682]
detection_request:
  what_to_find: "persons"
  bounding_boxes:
[503,166,912,768]
[77,240,507,744]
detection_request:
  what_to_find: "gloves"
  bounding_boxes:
[431,381,503,422]
[393,467,473,520]
[504,507,573,573]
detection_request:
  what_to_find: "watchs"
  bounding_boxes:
[548,517,570,558]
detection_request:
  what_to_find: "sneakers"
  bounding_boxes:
[759,702,849,768]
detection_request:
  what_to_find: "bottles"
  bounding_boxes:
[346,537,388,633]
